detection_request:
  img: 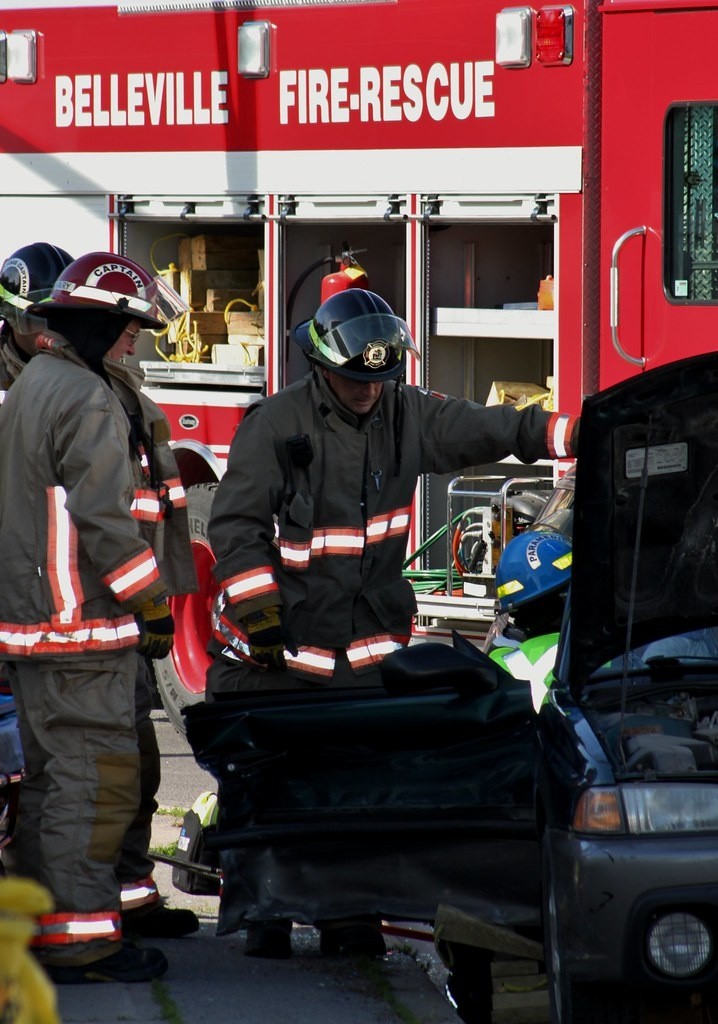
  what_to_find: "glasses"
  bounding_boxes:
[124,328,141,343]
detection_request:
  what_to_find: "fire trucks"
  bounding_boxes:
[0,0,717,648]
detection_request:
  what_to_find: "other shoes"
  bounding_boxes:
[243,920,295,960]
[122,907,200,938]
[45,941,169,986]
[320,926,387,961]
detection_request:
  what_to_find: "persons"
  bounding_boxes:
[0,243,198,985]
[215,288,579,683]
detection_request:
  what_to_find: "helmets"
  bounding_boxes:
[0,242,76,322]
[27,251,168,330]
[496,530,572,612]
[294,288,406,383]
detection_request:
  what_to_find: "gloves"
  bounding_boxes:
[133,593,175,659]
[240,606,298,672]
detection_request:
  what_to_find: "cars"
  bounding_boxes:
[180,350,718,1024]
